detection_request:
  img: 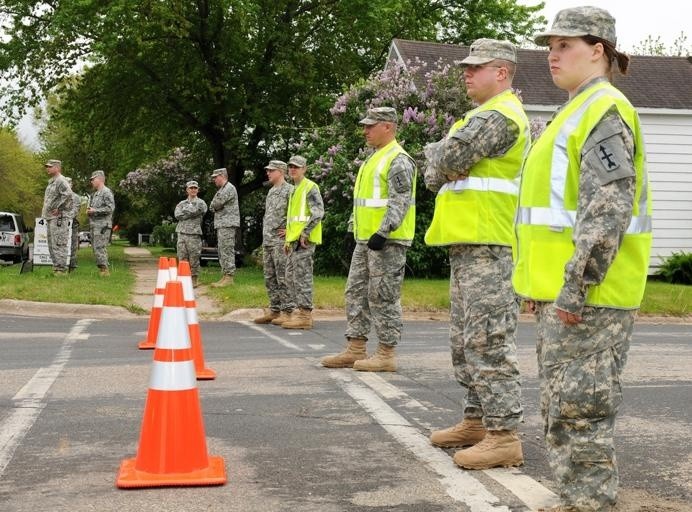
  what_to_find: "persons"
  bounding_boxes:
[320,106,417,372]
[283,155,324,330]
[209,167,241,288]
[86,170,116,277]
[256,158,295,325]
[174,181,208,289]
[424,35,531,470]
[511,7,654,511]
[41,158,72,278]
[66,175,81,269]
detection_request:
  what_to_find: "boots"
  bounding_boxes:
[55,270,67,276]
[212,275,234,288]
[320,339,367,368]
[98,266,109,277]
[191,276,198,287]
[255,311,281,323]
[453,430,524,470]
[353,342,397,371]
[282,308,312,330]
[272,310,292,325]
[431,418,487,448]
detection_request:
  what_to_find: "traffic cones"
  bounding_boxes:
[176,260,216,379]
[115,281,227,489]
[138,257,170,350]
[169,257,178,281]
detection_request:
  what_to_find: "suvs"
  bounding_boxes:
[0,211,29,265]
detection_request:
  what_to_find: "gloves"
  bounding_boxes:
[367,233,387,250]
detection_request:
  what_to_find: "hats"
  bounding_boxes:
[90,170,105,180]
[65,177,72,182]
[265,160,288,171]
[211,168,227,177]
[45,160,61,166]
[534,6,617,46]
[458,37,517,67]
[288,156,307,168]
[186,181,198,188]
[359,106,398,125]
